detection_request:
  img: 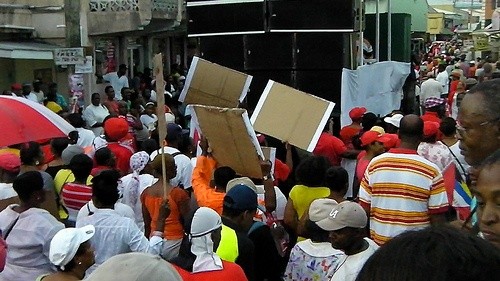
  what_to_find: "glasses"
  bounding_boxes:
[456,117,500,135]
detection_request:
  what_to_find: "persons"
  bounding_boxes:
[189,107,380,281]
[0,63,198,281]
[360,42,500,281]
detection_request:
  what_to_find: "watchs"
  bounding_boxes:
[262,175,271,181]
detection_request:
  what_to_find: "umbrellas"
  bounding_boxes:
[0,95,79,148]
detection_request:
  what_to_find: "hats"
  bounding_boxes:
[421,121,440,136]
[49,225,95,270]
[309,199,338,221]
[105,118,129,140]
[349,107,366,120]
[226,177,264,194]
[464,78,477,84]
[360,126,400,148]
[452,72,461,78]
[119,64,128,69]
[384,114,403,128]
[425,97,445,109]
[223,184,258,209]
[315,201,367,230]
